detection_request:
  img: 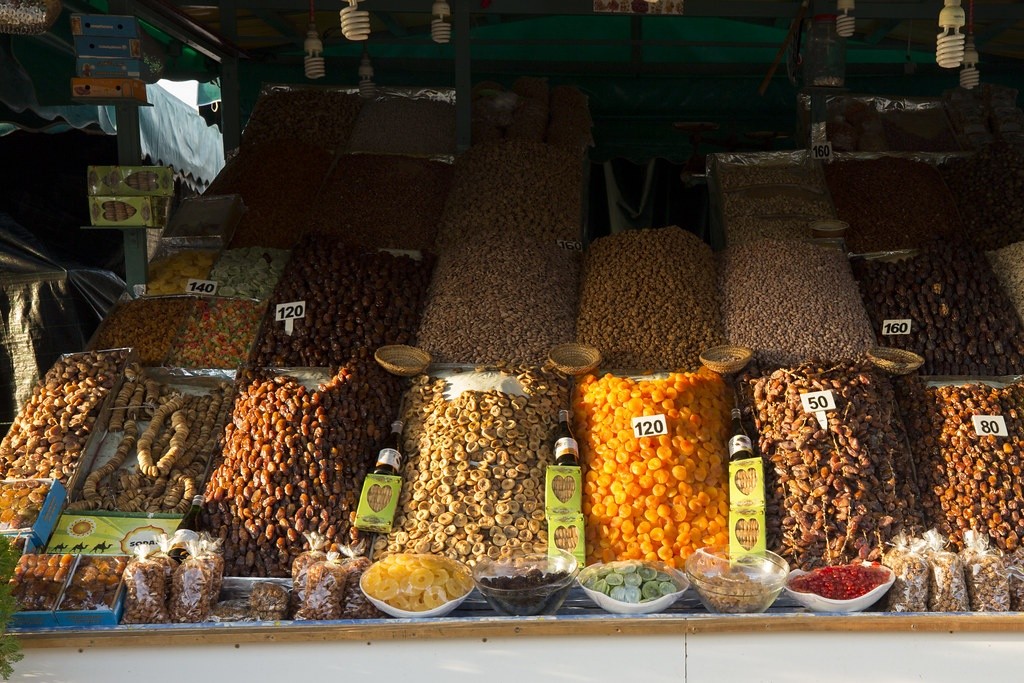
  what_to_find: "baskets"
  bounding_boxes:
[375,344,431,377]
[867,345,924,375]
[548,343,602,375]
[810,219,850,231]
[699,344,753,374]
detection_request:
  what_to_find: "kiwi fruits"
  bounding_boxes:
[580,560,676,605]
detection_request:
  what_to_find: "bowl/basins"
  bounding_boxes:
[782,564,896,612]
[685,544,790,613]
[359,560,475,619]
[472,545,579,615]
[576,563,690,613]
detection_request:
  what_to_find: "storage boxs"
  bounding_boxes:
[70,11,149,102]
[161,192,244,250]
[1,480,130,626]
[88,162,172,226]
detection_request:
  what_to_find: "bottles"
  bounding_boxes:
[167,494,206,566]
[802,13,848,87]
[373,420,405,476]
[727,408,754,461]
[553,410,579,466]
